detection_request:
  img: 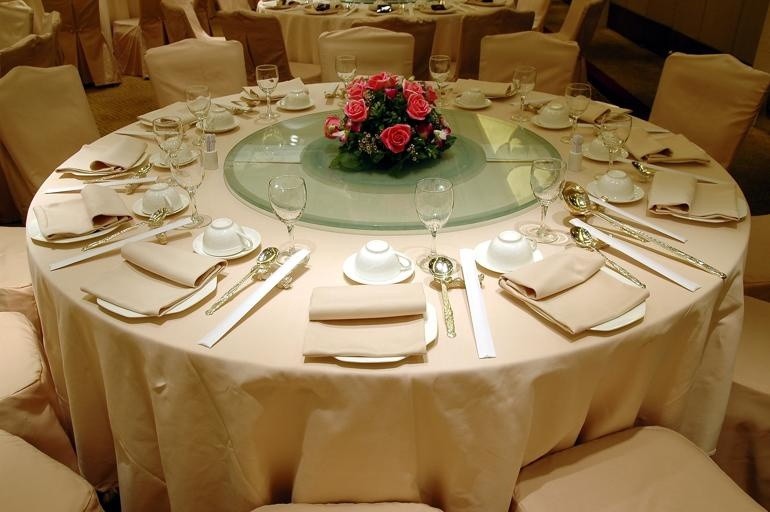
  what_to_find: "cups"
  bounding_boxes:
[160,147,193,165]
[598,169,633,200]
[357,239,413,279]
[206,108,234,130]
[460,88,488,105]
[142,183,184,214]
[487,230,538,271]
[283,89,313,108]
[540,102,569,127]
[589,133,622,159]
[202,218,254,257]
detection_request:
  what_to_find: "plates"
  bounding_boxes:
[582,146,629,162]
[71,151,148,177]
[28,210,121,244]
[342,252,415,286]
[532,115,573,130]
[587,181,645,204]
[453,98,492,111]
[473,239,543,274]
[333,302,438,364]
[193,226,262,261]
[149,151,199,169]
[584,299,647,333]
[195,117,241,134]
[96,276,219,318]
[261,0,508,19]
[133,195,191,218]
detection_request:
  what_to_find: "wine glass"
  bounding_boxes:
[256,65,280,125]
[511,65,539,121]
[429,53,454,99]
[153,116,181,187]
[267,175,310,265]
[167,147,211,229]
[524,157,568,243]
[183,84,215,146]
[592,112,632,180]
[563,82,592,143]
[335,54,356,98]
[414,177,460,274]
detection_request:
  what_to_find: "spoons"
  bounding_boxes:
[558,179,728,283]
[430,257,457,338]
[632,161,718,183]
[569,226,647,290]
[204,247,281,316]
[80,207,167,251]
[83,162,153,183]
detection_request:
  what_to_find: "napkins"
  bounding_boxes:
[498,247,650,340]
[647,172,740,222]
[81,240,228,318]
[302,283,426,357]
[617,127,711,165]
[566,95,632,124]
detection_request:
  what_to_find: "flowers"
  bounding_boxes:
[323,71,457,179]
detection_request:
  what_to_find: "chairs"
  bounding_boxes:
[743,215,770,303]
[251,502,442,512]
[0,0,414,225]
[415,0,607,97]
[0,224,104,512]
[710,295,770,512]
[510,426,768,512]
[649,52,770,170]
